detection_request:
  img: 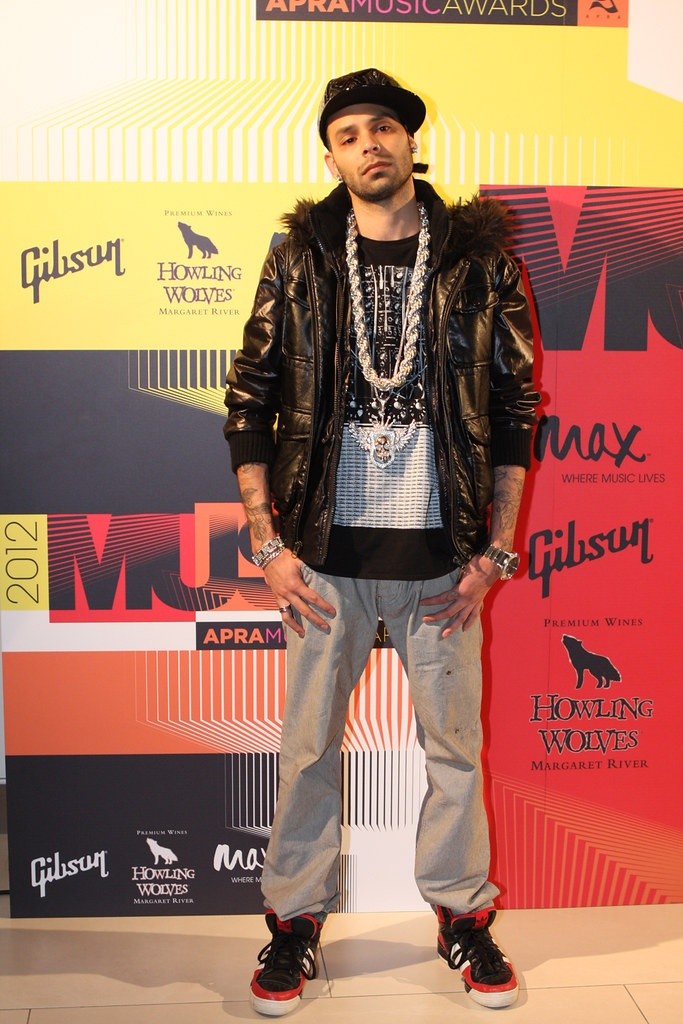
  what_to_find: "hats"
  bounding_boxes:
[318,68,427,153]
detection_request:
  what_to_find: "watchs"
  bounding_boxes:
[479,543,520,581]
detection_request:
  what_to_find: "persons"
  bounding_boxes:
[223,69,541,1018]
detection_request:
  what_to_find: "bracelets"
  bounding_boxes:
[252,537,285,568]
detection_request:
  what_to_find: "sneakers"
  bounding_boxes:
[250,906,323,1015]
[434,901,518,1008]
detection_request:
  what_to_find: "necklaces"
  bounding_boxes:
[348,291,417,467]
[341,201,428,393]
